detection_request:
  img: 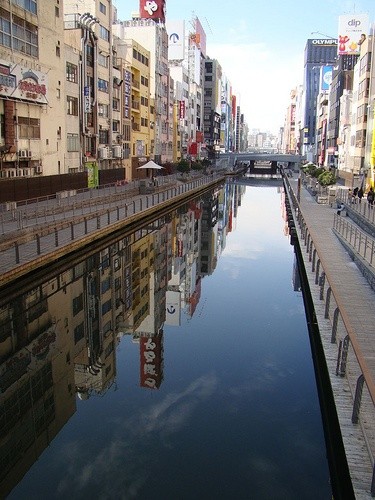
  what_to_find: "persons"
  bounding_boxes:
[349,189,358,204]
[367,188,375,209]
[353,187,359,195]
[205,158,293,180]
[358,189,363,203]
[337,203,345,215]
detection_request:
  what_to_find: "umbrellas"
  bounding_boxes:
[135,161,165,179]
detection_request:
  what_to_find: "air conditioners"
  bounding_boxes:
[100,147,110,159]
[30,167,35,176]
[10,168,16,177]
[20,150,26,157]
[23,167,30,176]
[114,145,122,158]
[2,168,9,177]
[28,150,32,157]
[35,166,43,172]
[16,168,23,176]
